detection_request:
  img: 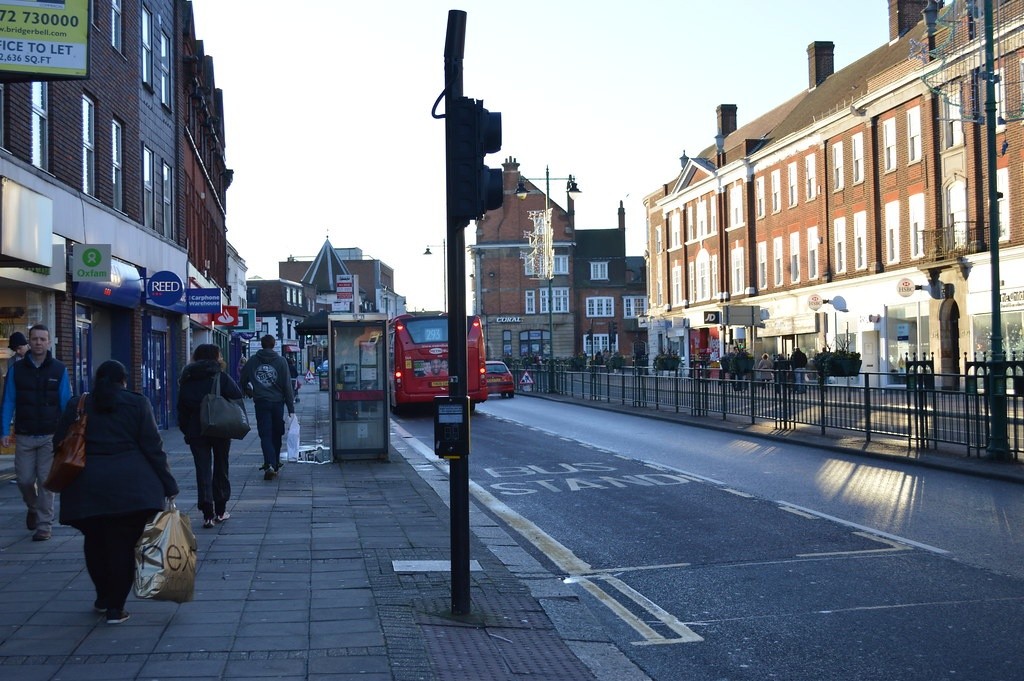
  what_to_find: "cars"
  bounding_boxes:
[317,360,329,374]
[485,360,515,399]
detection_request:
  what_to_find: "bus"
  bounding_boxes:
[388,309,490,415]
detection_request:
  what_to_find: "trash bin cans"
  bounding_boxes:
[319,371,329,391]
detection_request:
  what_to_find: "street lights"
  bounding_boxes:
[394,295,407,316]
[512,164,583,394]
[422,237,447,313]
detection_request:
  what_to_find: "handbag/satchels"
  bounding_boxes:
[200,372,251,441]
[43,391,87,493]
[134,497,196,603]
[285,414,301,462]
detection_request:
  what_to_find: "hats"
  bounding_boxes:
[8,332,28,348]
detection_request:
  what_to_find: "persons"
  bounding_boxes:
[418,357,448,377]
[571,350,587,371]
[791,347,807,393]
[289,357,300,404]
[595,348,622,374]
[522,352,542,369]
[8,332,30,371]
[734,346,739,352]
[51,359,181,623]
[237,356,246,378]
[176,344,244,530]
[240,335,295,480]
[1,324,72,540]
[757,353,772,390]
[503,353,512,369]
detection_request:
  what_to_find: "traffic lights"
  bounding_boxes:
[586,328,592,340]
[460,95,506,227]
[611,320,618,337]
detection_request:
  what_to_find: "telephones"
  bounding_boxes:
[337,363,358,384]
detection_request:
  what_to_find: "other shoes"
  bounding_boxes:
[26,509,37,530]
[276,462,284,474]
[203,519,215,527]
[105,611,131,623]
[259,464,277,481]
[217,512,231,521]
[32,529,51,539]
[94,599,107,612]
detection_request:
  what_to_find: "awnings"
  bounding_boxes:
[285,346,300,353]
